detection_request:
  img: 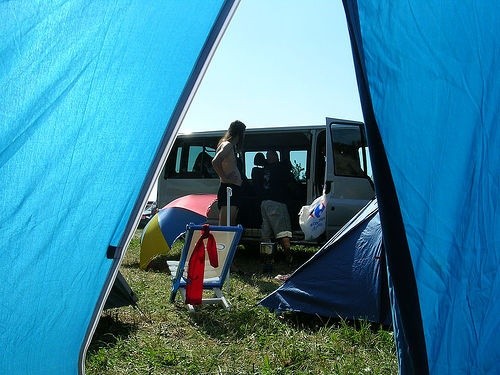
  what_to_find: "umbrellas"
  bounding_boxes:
[140,192,219,270]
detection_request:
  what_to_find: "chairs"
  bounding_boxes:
[165,223,243,311]
[252,152,267,181]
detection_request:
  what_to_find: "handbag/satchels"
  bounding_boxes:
[298,193,327,240]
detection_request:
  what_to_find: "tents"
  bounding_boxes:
[254,191,393,329]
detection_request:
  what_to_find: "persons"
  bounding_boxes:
[258,157,302,270]
[212,119,246,274]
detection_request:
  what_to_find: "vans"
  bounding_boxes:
[156,117,376,253]
